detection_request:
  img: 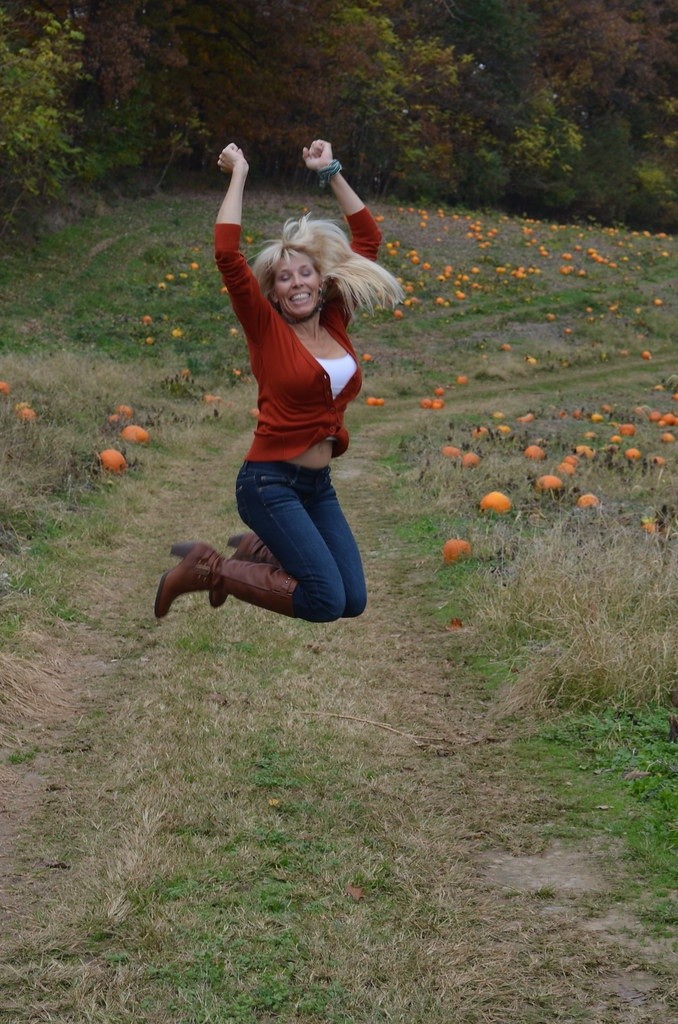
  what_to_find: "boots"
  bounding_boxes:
[209,534,288,608]
[155,542,299,618]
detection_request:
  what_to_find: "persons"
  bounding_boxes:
[154,139,383,624]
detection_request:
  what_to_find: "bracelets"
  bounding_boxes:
[316,159,342,189]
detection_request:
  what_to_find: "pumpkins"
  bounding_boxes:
[443,538,471,565]
[0,235,260,475]
[364,206,678,533]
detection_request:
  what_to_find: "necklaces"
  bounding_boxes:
[282,308,319,325]
